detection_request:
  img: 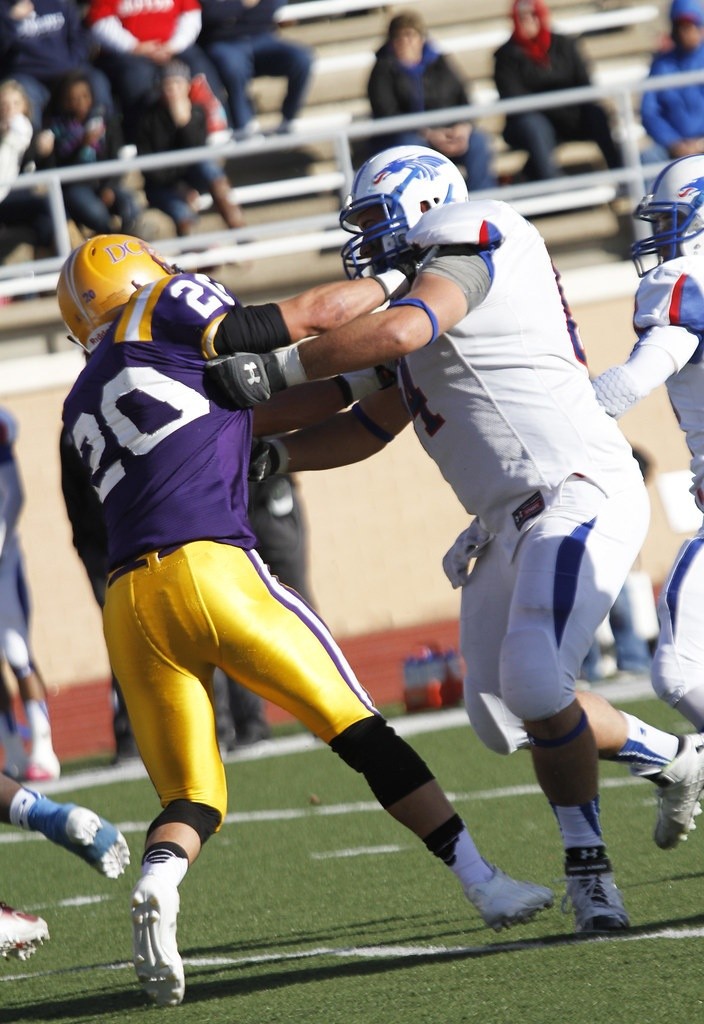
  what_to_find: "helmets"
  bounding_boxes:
[57,234,188,356]
[339,143,471,286]
[629,151,704,277]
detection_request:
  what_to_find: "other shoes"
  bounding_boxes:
[2,748,61,784]
[238,718,271,747]
[219,741,235,755]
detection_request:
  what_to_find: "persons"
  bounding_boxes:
[1,1,704,960]
[55,234,552,1010]
[203,143,703,929]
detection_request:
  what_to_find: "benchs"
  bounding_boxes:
[0,0,704,298]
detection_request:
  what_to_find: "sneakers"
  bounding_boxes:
[1,901,52,963]
[465,863,555,933]
[567,869,631,930]
[131,857,189,1009]
[630,731,704,850]
[27,794,130,881]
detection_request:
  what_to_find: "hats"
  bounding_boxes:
[670,0,704,45]
[389,14,426,37]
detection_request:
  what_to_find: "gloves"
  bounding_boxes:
[397,243,440,290]
[247,436,282,483]
[205,349,289,411]
